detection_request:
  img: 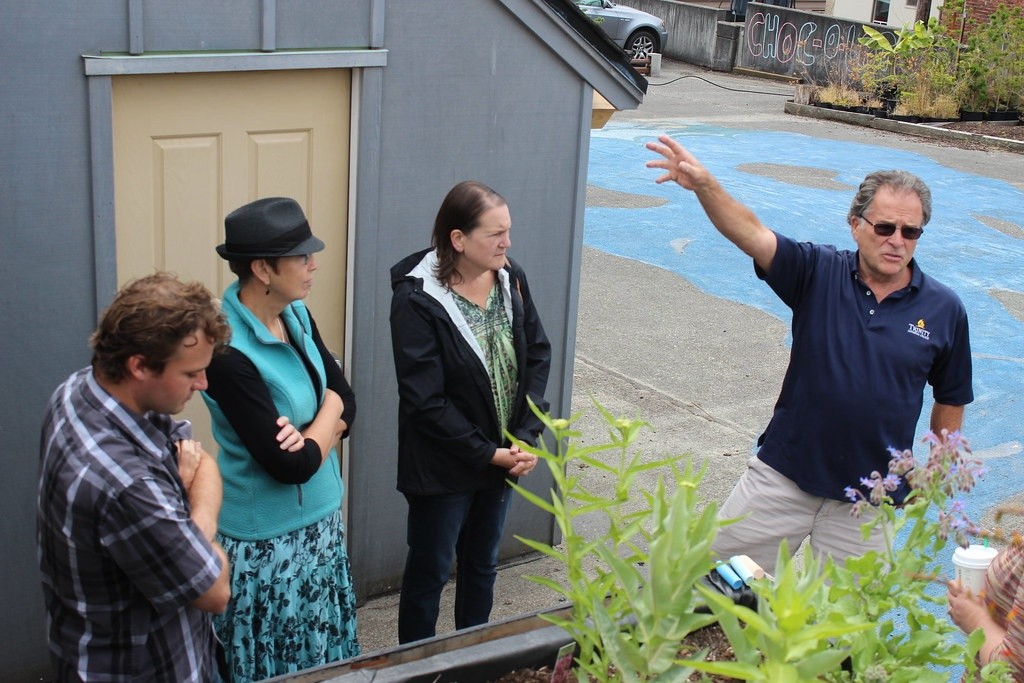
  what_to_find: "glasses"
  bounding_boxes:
[857,213,924,240]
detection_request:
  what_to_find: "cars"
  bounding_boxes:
[575,0,666,62]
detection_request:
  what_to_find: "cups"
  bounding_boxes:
[952,545,999,602]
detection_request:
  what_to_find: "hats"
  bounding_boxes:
[215,197,326,262]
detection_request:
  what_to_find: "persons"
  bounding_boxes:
[390,181,552,644]
[644,136,974,601]
[36,276,232,683]
[199,198,356,683]
[947,537,1024,683]
